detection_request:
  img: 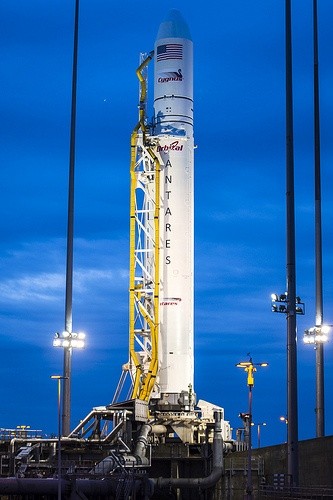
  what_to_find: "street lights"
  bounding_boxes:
[280,416,288,442]
[251,421,267,449]
[50,374,70,500]
